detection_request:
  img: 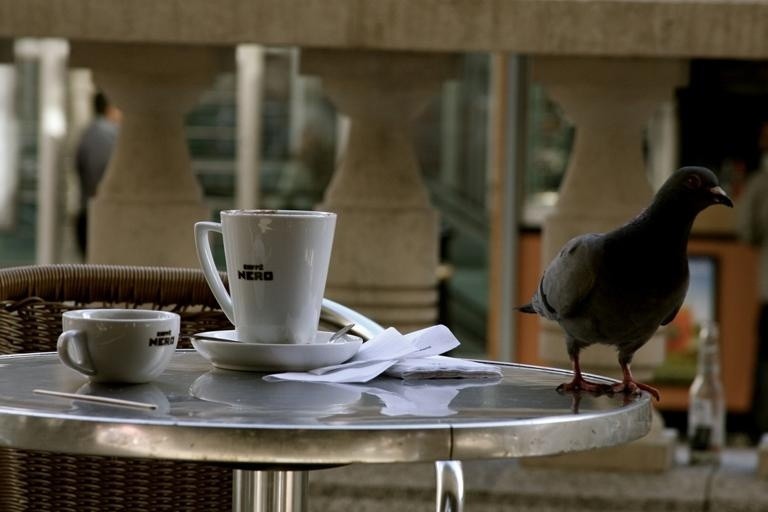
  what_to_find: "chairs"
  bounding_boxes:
[2,263,466,512]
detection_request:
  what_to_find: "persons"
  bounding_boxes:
[742,159,768,446]
[75,92,120,258]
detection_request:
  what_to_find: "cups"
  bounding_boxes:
[194,208,338,344]
[55,310,182,383]
[74,384,171,417]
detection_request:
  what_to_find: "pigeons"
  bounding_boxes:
[511,166,734,402]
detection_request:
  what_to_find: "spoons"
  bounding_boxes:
[194,324,356,346]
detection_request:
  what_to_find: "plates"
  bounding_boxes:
[190,327,363,372]
[188,373,363,412]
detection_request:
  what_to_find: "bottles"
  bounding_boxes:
[688,323,724,466]
[757,434,767,479]
